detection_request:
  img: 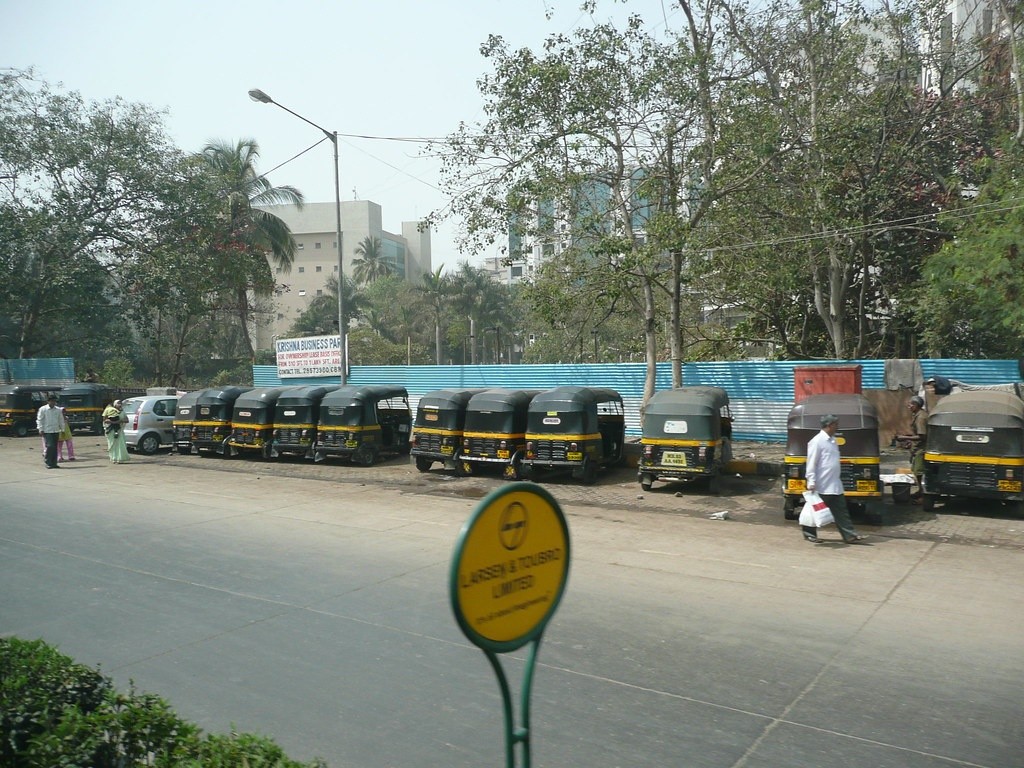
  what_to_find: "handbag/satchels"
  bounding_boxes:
[798,502,817,527]
[802,490,835,528]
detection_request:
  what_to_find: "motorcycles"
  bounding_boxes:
[523,385,627,485]
[191,386,251,458]
[455,388,548,483]
[921,389,1024,515]
[780,394,885,526]
[412,388,489,479]
[638,384,735,497]
[312,385,413,470]
[272,384,341,464]
[228,386,298,464]
[175,389,221,454]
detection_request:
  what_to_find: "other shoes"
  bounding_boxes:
[114,432,118,438]
[847,534,869,544]
[70,458,75,461]
[60,458,65,463]
[106,430,109,434]
[45,465,59,469]
[806,536,823,543]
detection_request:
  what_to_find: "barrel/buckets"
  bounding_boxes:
[892,482,910,502]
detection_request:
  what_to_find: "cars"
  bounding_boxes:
[55,382,115,437]
[0,383,69,439]
[119,394,184,455]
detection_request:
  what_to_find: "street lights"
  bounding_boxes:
[247,87,348,386]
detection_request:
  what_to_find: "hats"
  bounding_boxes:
[114,400,120,406]
[911,396,924,407]
[820,414,838,428]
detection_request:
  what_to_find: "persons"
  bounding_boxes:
[895,396,929,503]
[101,398,130,465]
[36,394,75,469]
[801,415,869,545]
